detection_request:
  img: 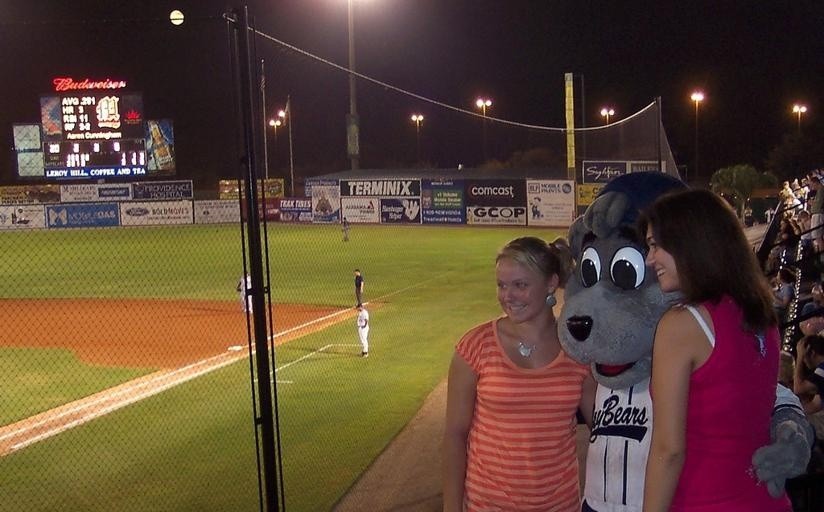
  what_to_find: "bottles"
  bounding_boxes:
[147,119,175,170]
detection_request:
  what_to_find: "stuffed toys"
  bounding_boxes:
[546,171,814,511]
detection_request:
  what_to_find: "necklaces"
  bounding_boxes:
[511,317,553,361]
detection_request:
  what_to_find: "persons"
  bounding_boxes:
[357,303,369,357]
[638,189,792,512]
[353,269,365,310]
[736,168,823,511]
[440,235,596,511]
[342,218,351,241]
[237,271,252,313]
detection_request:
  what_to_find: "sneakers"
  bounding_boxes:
[358,352,367,358]
